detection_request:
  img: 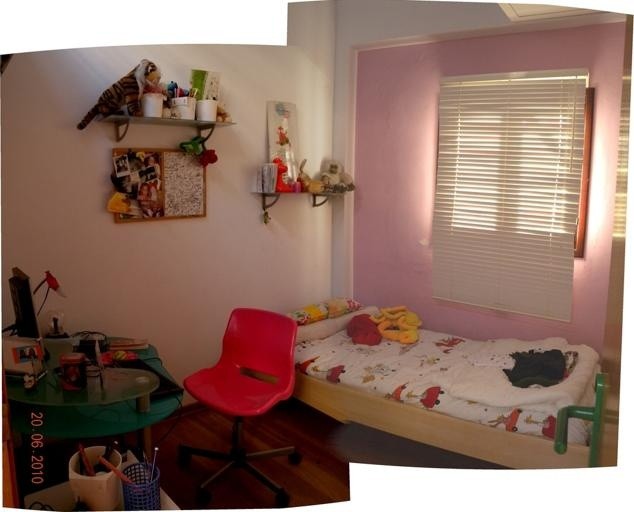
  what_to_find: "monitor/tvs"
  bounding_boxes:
[9,267,39,338]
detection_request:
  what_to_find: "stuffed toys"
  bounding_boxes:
[321,161,355,193]
[347,313,382,347]
[368,306,422,345]
[501,348,566,389]
[74,58,162,132]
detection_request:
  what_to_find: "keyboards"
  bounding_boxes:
[113,358,183,402]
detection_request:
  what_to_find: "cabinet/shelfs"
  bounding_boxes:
[96,112,339,210]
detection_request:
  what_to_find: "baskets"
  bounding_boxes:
[121,462,162,509]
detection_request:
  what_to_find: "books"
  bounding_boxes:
[108,337,150,351]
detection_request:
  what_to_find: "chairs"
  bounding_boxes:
[174,307,301,504]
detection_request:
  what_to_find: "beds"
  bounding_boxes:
[274,294,610,470]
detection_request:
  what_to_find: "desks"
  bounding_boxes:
[22,447,191,511]
[3,339,183,489]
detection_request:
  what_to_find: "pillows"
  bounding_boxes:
[284,295,326,324]
[322,295,363,320]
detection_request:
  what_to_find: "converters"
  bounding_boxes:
[79,340,104,353]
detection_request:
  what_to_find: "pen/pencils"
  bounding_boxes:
[143,447,159,496]
[96,455,144,497]
[189,88,197,97]
[103,441,119,460]
[170,81,179,98]
[79,443,95,476]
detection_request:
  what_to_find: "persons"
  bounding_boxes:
[115,154,163,216]
